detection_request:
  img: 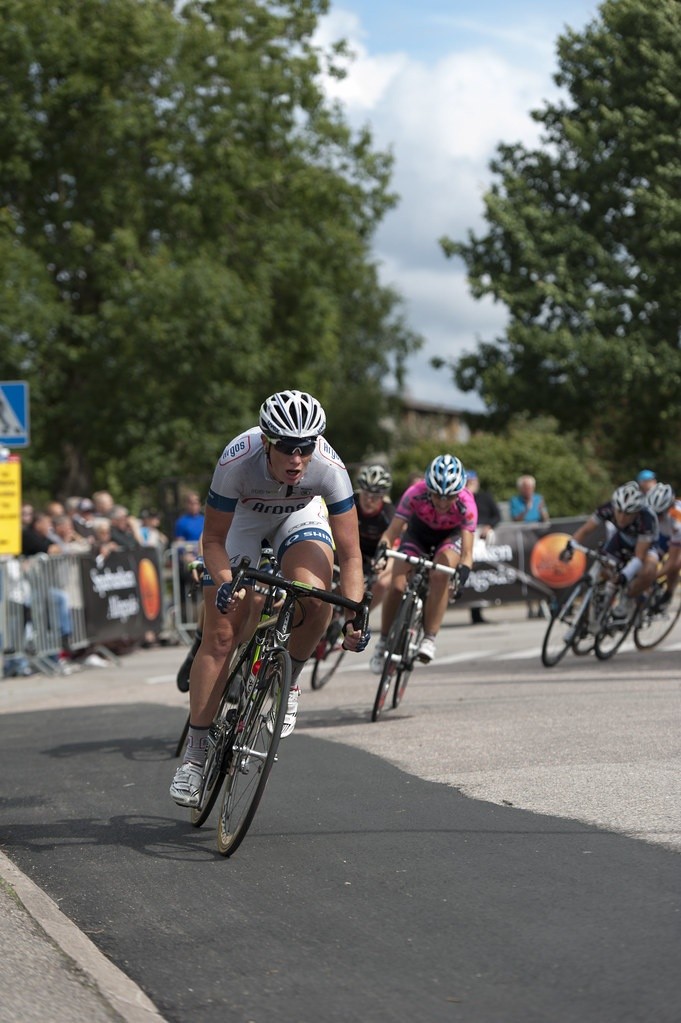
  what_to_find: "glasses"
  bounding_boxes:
[434,493,457,502]
[264,433,316,457]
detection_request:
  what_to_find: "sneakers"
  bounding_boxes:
[169,759,207,808]
[610,589,633,620]
[369,638,389,675]
[266,684,301,739]
[415,637,436,665]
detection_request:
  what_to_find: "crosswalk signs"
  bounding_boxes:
[0,380,29,448]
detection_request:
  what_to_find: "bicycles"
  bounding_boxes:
[541,539,681,668]
[173,540,464,857]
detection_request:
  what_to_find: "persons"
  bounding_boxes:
[560,470,681,614]
[171,391,370,816]
[509,475,549,522]
[332,465,395,649]
[368,453,502,674]
[0,489,173,664]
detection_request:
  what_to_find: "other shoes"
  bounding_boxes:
[653,589,676,613]
[176,649,194,693]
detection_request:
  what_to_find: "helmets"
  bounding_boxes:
[611,481,645,514]
[424,453,468,497]
[636,469,656,484]
[356,463,393,496]
[258,389,328,448]
[644,481,676,515]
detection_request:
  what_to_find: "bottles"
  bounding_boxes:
[596,586,606,608]
[225,661,248,704]
[236,659,266,717]
